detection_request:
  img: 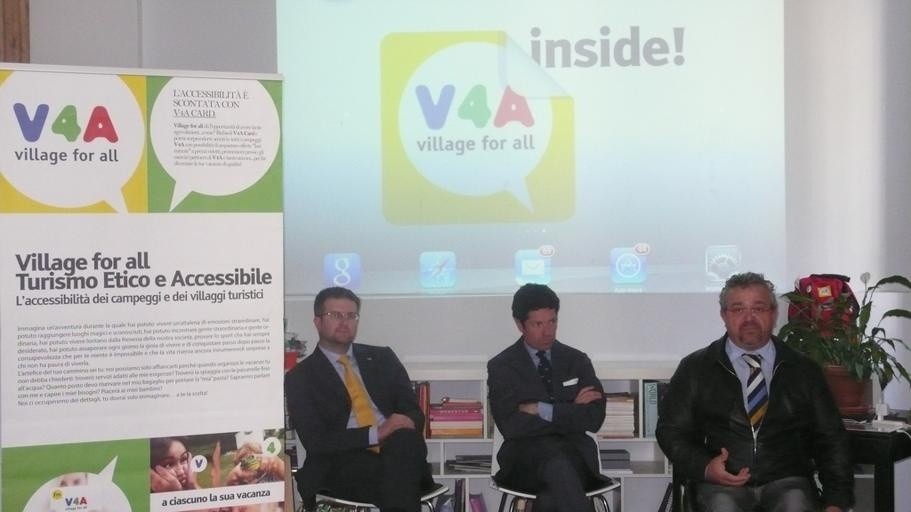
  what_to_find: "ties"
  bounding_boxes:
[336,354,381,454]
[740,353,771,438]
[534,351,556,404]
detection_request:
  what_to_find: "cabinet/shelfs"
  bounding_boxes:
[403,366,691,478]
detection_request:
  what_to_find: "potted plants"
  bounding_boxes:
[772,269,911,423]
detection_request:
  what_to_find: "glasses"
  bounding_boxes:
[318,310,361,321]
[725,305,773,315]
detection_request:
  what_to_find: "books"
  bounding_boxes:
[411,381,483,439]
[446,454,492,474]
[596,391,635,439]
[600,449,633,475]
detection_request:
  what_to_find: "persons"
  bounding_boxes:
[486,280,612,511]
[219,442,285,511]
[284,285,435,512]
[150,438,223,492]
[150,437,188,487]
[654,271,857,512]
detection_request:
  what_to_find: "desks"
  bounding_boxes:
[829,412,911,512]
